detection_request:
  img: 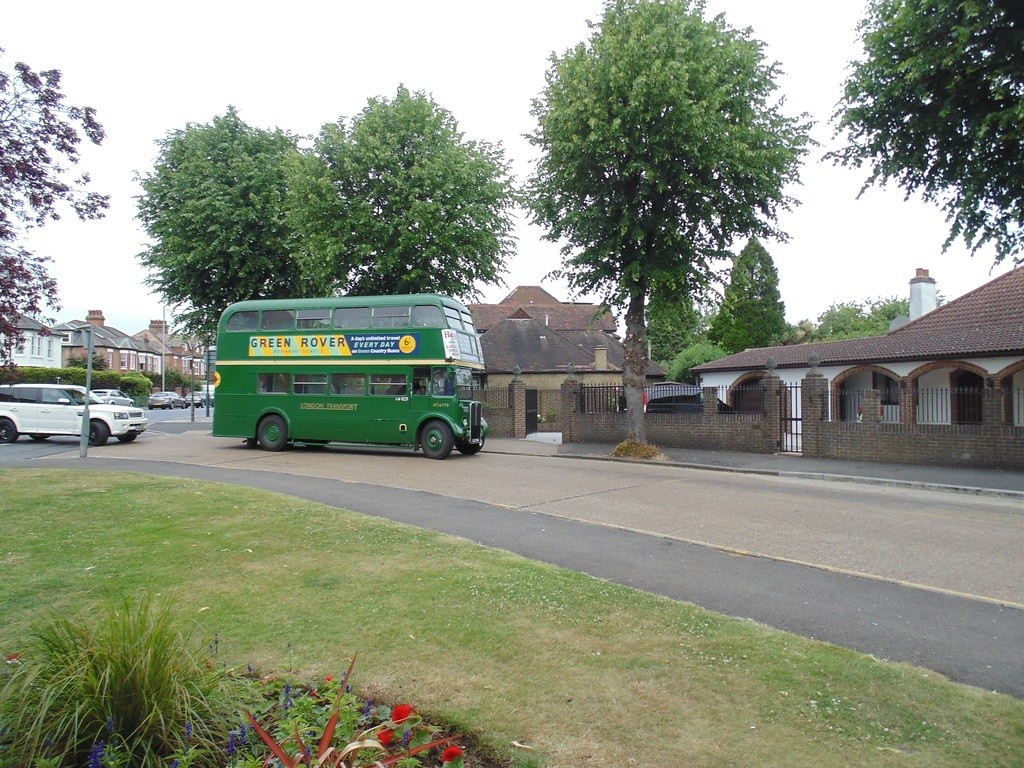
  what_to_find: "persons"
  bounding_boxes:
[427,369,445,395]
[385,377,402,395]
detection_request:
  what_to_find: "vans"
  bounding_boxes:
[642,381,733,412]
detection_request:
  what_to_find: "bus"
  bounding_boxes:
[211,292,489,460]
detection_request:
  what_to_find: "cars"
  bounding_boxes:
[148,391,186,410]
[184,391,214,408]
[82,389,134,407]
[0,384,148,447]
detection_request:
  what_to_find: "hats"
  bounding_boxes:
[433,370,440,375]
